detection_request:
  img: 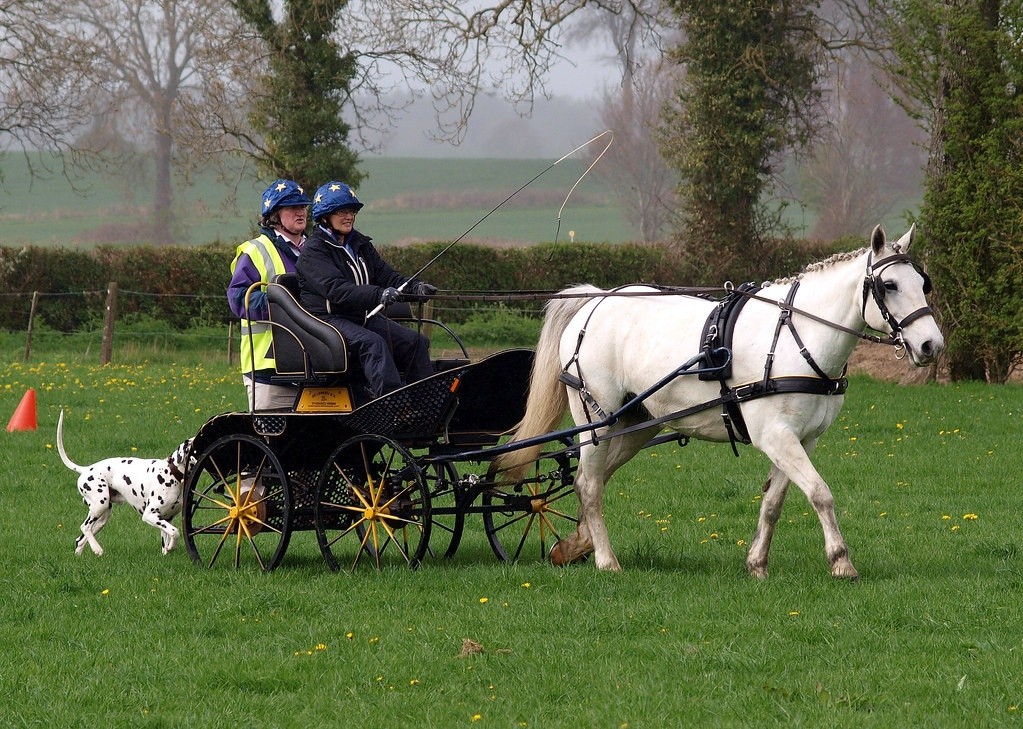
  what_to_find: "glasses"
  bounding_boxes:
[325,209,358,216]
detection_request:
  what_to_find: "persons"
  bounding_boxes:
[226,179,438,496]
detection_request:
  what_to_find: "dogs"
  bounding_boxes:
[56,407,207,557]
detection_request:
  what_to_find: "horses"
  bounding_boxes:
[480,223,945,580]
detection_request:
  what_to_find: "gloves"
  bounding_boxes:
[380,287,402,305]
[416,282,437,301]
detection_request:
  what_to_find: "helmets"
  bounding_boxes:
[262,177,313,216]
[313,181,364,220]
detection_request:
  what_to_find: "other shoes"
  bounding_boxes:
[292,496,321,508]
[395,408,427,425]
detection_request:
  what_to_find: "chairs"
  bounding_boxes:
[259,266,431,409]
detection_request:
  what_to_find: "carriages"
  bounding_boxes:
[177,221,946,584]
